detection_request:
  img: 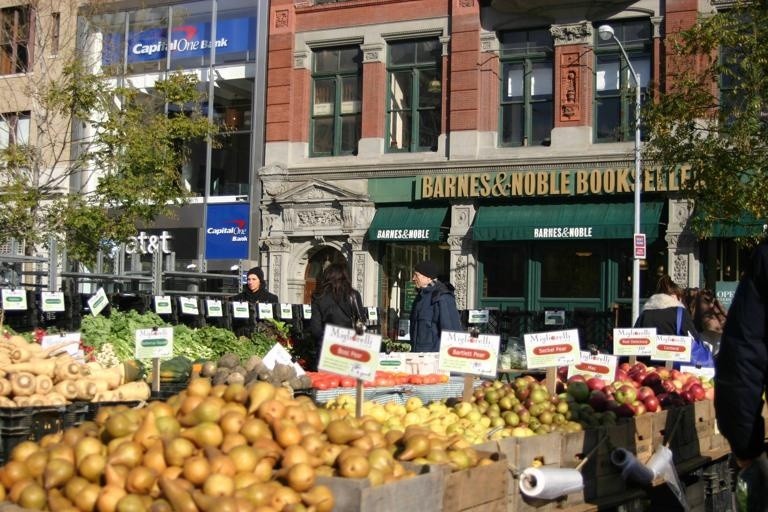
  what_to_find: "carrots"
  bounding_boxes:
[0,335,151,406]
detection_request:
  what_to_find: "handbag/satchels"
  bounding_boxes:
[676,334,715,369]
[351,313,366,334]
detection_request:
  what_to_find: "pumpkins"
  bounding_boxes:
[125,356,208,384]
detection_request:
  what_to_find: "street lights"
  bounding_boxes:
[598,24,641,332]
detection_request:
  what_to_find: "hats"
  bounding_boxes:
[415,260,439,279]
[248,267,264,281]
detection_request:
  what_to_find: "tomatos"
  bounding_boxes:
[305,369,448,390]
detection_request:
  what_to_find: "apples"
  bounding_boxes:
[447,362,715,438]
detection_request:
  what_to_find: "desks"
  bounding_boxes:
[313,376,508,406]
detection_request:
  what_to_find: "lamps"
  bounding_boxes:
[428,70,442,94]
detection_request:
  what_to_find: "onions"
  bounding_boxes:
[201,354,312,391]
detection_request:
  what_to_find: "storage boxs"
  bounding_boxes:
[317,399,723,512]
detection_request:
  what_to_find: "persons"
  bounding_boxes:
[307,261,367,369]
[408,260,465,352]
[635,273,698,367]
[714,241,768,512]
[232,266,279,303]
